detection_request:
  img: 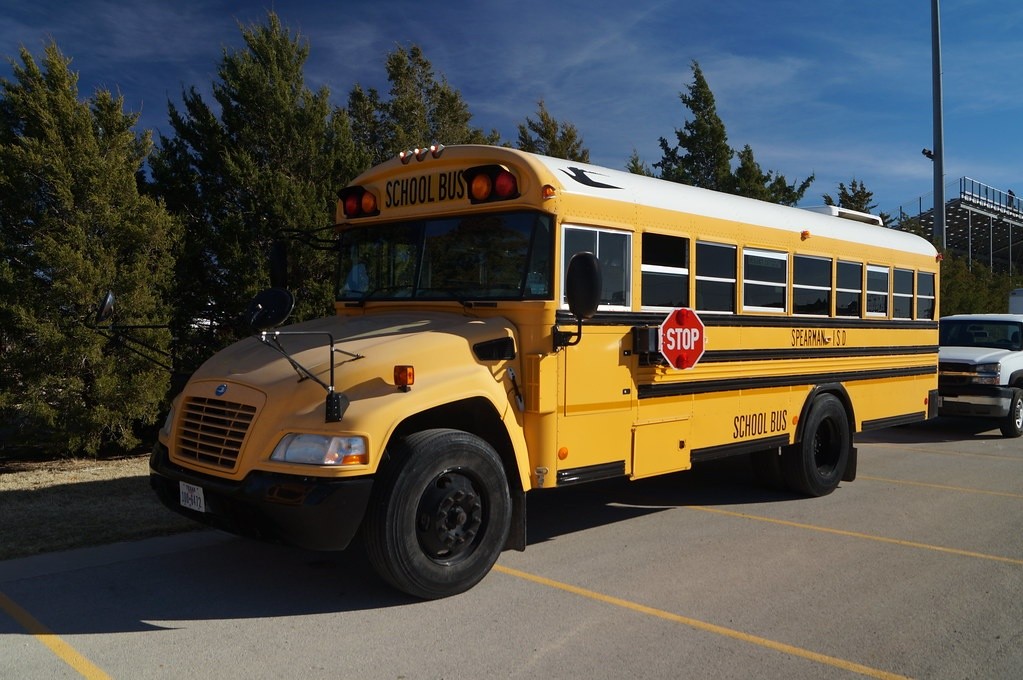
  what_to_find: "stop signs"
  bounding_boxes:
[660,308,706,370]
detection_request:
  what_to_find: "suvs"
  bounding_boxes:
[939,314,1023,439]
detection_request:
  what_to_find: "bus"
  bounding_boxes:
[84,143,942,605]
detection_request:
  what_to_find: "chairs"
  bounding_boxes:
[953,333,976,346]
[1011,332,1021,344]
